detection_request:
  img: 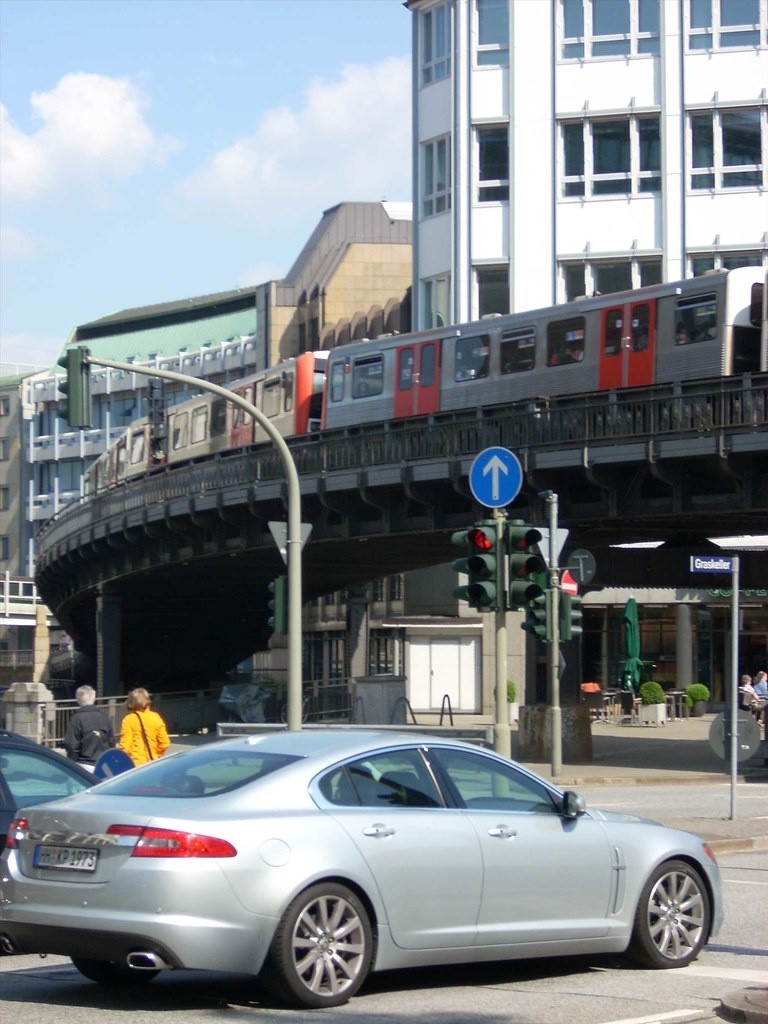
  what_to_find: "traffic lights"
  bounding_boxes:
[268,575,288,640]
[451,518,540,615]
[58,345,96,432]
[533,588,583,647]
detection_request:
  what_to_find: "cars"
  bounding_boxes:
[0,732,103,954]
[0,727,722,1008]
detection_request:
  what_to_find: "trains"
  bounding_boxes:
[81,266,768,503]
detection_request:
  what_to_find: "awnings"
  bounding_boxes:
[580,584,768,609]
[609,530,768,554]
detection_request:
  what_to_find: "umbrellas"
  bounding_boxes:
[621,595,644,723]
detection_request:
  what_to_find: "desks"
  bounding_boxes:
[666,691,685,719]
[603,692,617,722]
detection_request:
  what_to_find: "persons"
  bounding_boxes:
[64,685,116,774]
[353,382,370,398]
[738,674,760,712]
[676,320,717,346]
[115,688,170,768]
[455,359,488,382]
[561,345,576,365]
[753,671,768,726]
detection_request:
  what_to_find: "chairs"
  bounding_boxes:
[335,765,429,808]
[583,687,688,724]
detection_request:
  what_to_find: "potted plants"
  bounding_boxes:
[638,682,667,727]
[684,683,710,718]
